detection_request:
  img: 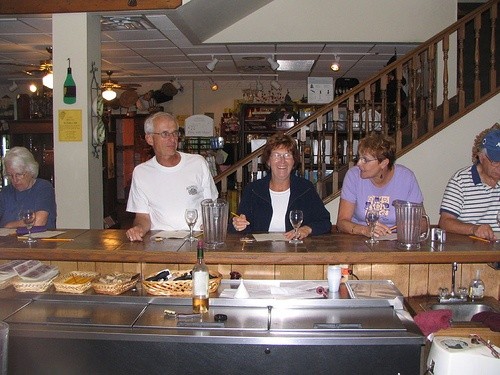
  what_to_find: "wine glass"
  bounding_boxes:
[365,205,378,246]
[22,209,36,245]
[494,209,500,249]
[184,208,199,242]
[289,209,304,245]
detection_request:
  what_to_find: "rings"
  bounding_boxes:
[298,233,301,235]
[129,236,133,239]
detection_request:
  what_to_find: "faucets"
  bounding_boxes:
[452,262,457,295]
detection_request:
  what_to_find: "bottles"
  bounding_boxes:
[192,240,209,313]
[63,59,76,103]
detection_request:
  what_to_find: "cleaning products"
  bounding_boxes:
[468,268,486,300]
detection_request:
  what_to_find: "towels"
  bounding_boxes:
[472,312,500,331]
[414,310,451,336]
[17,222,45,234]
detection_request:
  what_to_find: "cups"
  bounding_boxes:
[201,199,230,250]
[325,264,342,293]
[394,199,430,249]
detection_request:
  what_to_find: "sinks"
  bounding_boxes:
[429,304,496,322]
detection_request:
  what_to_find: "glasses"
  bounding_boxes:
[486,156,493,162]
[360,156,378,163]
[4,170,28,179]
[148,130,180,138]
[271,152,293,158]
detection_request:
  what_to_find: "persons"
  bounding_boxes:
[0,146,57,230]
[125,111,219,242]
[337,133,431,238]
[229,131,332,241]
[438,123,500,242]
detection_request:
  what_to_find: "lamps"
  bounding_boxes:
[44,46,53,89]
[102,72,120,101]
[267,55,279,71]
[271,77,279,89]
[7,79,21,96]
[206,55,218,73]
[167,75,186,95]
[331,56,340,71]
[203,77,221,93]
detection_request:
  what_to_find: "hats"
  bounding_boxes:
[480,130,500,161]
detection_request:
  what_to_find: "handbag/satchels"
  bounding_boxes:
[154,90,173,103]
[149,100,158,112]
[161,82,177,96]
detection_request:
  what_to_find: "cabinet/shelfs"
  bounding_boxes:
[240,105,384,185]
[101,111,158,204]
